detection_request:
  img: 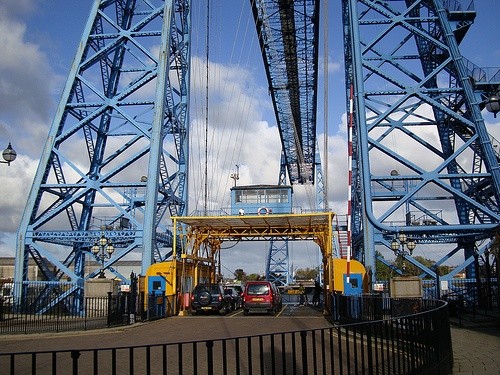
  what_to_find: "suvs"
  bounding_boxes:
[270,285,282,313]
[191,283,227,316]
[241,281,279,315]
[222,284,244,312]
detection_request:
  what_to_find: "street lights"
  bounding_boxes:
[390,229,415,275]
[92,235,115,279]
